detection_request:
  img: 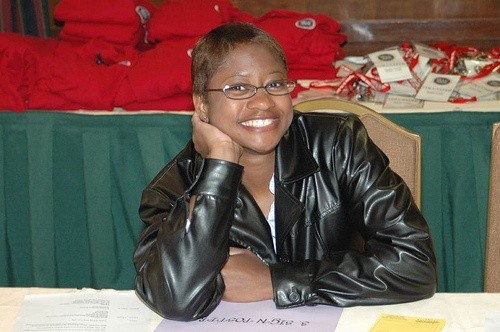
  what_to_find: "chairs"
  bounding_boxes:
[291,98,422,211]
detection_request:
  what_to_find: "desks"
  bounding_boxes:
[2,46,500,293]
[0,283,500,331]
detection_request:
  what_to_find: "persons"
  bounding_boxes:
[131,21,439,323]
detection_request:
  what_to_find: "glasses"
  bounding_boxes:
[201,77,297,101]
